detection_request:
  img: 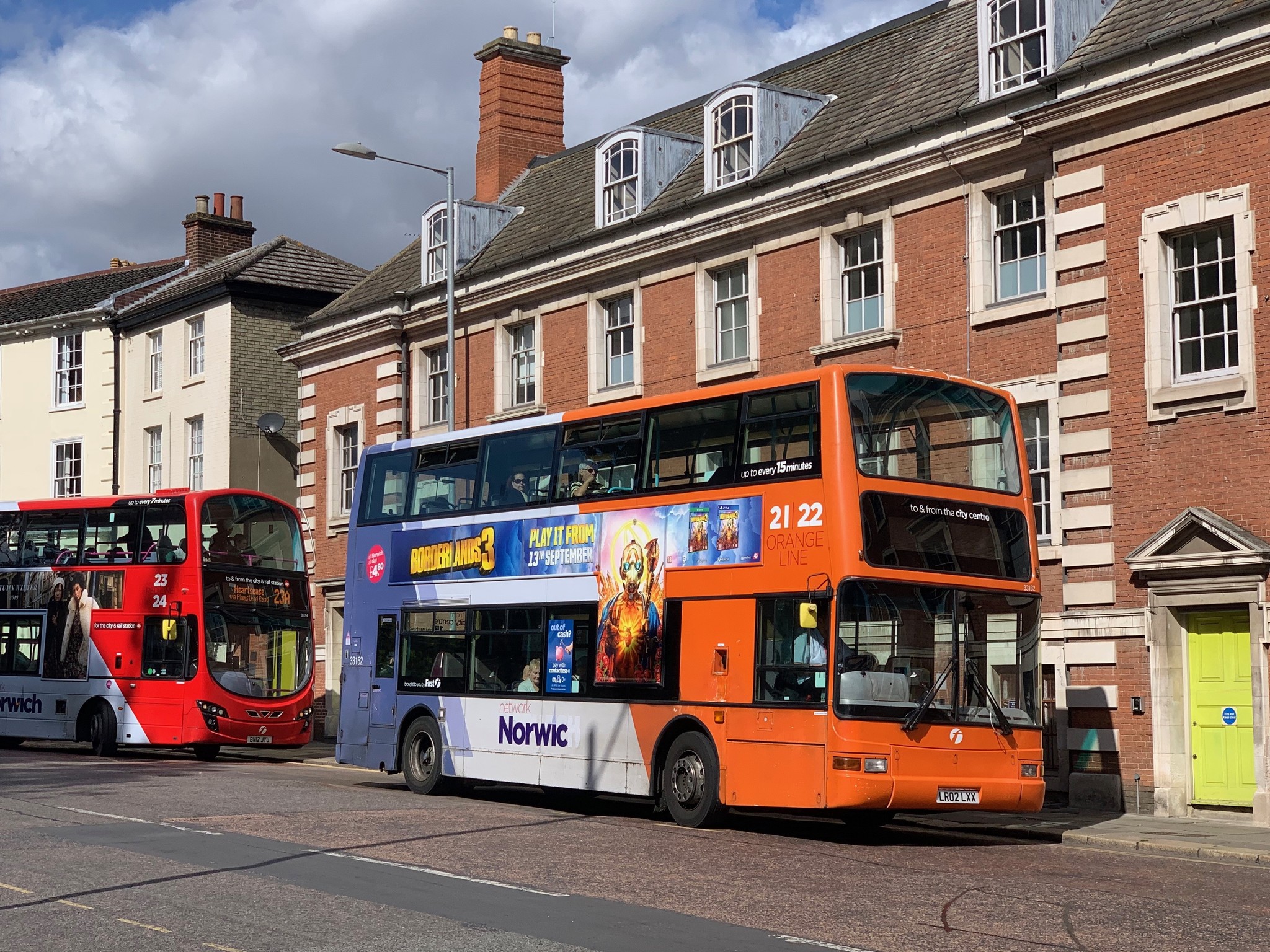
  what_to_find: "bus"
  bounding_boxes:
[334,361,1047,832]
[0,487,317,763]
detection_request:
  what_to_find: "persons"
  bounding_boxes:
[210,519,263,566]
[0,641,30,675]
[41,577,70,679]
[790,605,879,702]
[569,459,610,497]
[116,510,156,559]
[59,572,100,680]
[506,471,531,505]
[517,658,541,693]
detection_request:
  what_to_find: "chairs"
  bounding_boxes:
[418,472,660,513]
[1,536,187,563]
[405,639,525,694]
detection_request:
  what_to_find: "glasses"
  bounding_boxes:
[512,479,526,484]
[582,469,598,473]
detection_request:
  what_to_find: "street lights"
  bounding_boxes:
[332,140,455,511]
